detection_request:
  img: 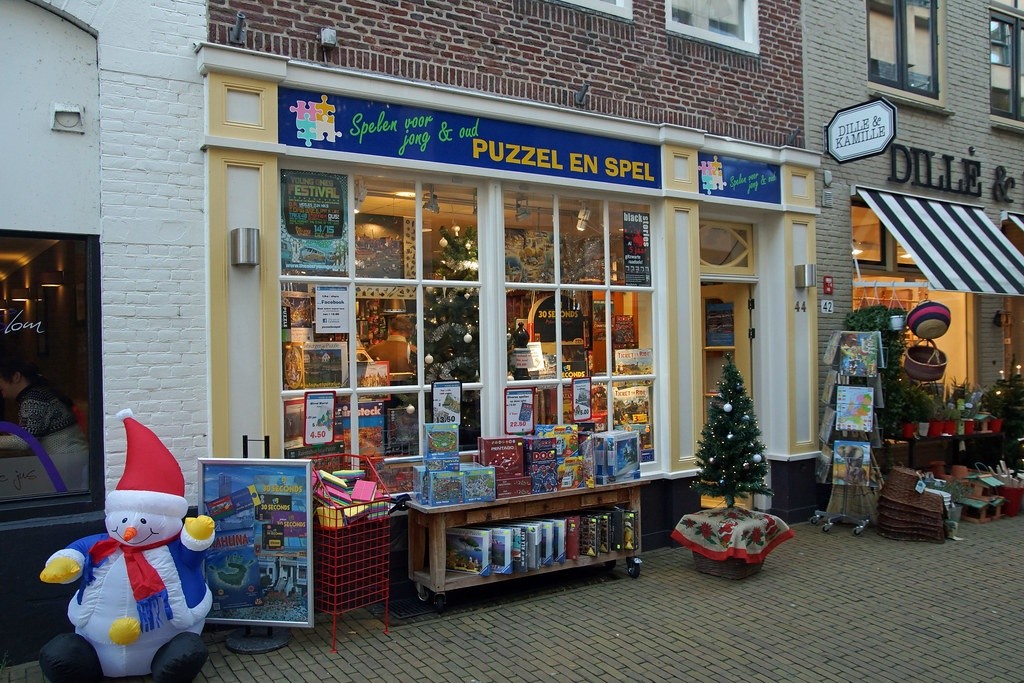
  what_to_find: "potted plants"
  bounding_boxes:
[896,383,976,440]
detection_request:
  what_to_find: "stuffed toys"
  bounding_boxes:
[40,408,214,683]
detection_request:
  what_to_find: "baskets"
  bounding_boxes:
[905,299,951,339]
[904,338,947,381]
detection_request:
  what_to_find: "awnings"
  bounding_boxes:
[854,185,1024,296]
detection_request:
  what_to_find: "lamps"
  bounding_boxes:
[422,183,440,215]
[576,199,593,232]
[514,195,529,222]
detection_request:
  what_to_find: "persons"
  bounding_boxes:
[0,351,90,456]
[367,317,417,372]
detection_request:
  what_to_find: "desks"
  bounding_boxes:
[403,478,653,616]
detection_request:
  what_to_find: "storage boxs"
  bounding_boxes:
[280,330,394,464]
[411,423,649,576]
[614,348,653,372]
